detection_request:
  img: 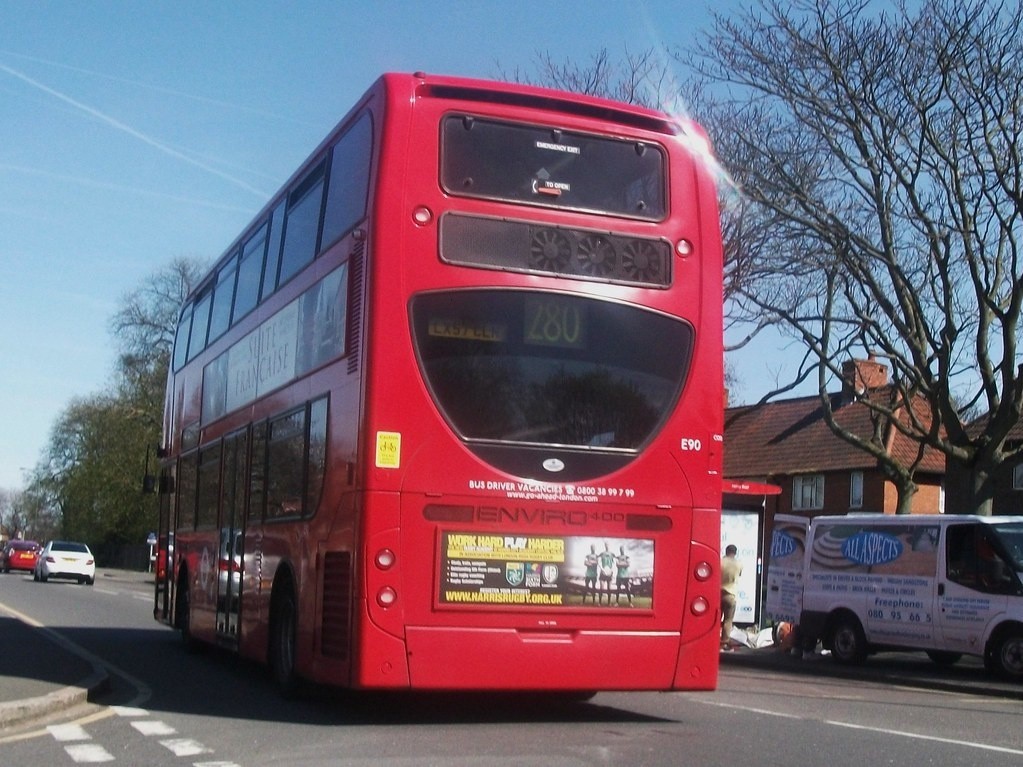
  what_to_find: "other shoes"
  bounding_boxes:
[791,647,801,655]
[720,643,733,653]
[802,650,822,661]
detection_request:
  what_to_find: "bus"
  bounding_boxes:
[143,68,727,708]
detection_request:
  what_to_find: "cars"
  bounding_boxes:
[0,540,42,575]
[33,539,96,586]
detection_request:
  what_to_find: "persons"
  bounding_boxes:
[582,544,599,605]
[720,544,742,650]
[615,545,635,608]
[596,542,616,607]
[790,626,824,660]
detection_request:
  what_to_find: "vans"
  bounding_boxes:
[797,512,1023,684]
